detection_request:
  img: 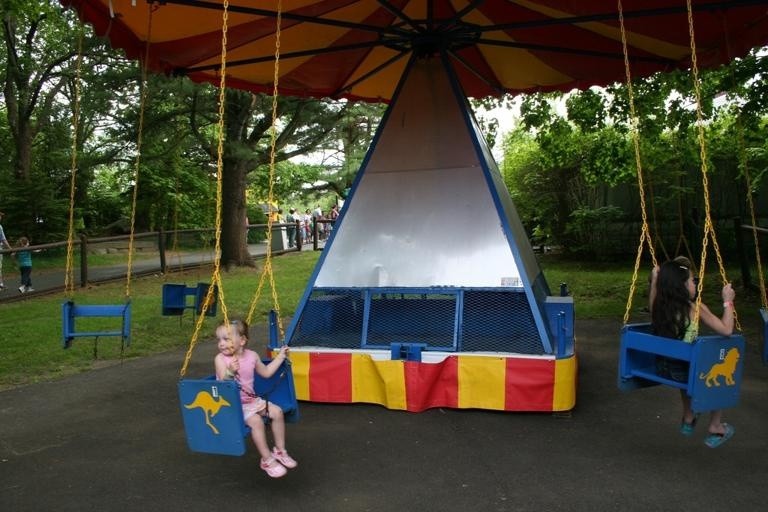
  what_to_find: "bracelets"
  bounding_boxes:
[225,368,236,378]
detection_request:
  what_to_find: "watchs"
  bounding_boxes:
[722,299,734,310]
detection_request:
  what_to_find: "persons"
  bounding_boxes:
[214,317,298,478]
[10,237,48,294]
[245,203,340,250]
[649,260,737,449]
[0,212,15,289]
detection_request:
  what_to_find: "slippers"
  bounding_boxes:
[704,423,736,449]
[680,410,700,434]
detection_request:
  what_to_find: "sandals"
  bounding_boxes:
[260,456,287,478]
[270,446,298,469]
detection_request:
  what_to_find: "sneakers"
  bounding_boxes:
[18,286,25,293]
[0,285,8,291]
[26,286,35,292]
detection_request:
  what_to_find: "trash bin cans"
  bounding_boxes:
[271,220,288,252]
